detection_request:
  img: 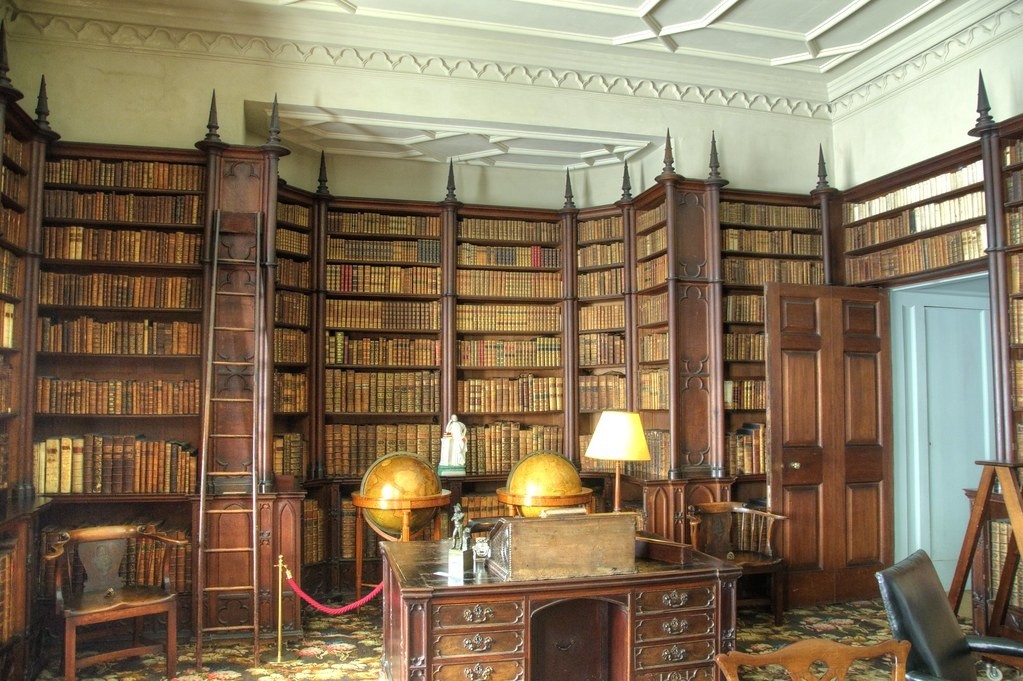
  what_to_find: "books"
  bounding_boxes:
[2,121,1023,614]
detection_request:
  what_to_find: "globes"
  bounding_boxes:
[496,450,593,517]
[351,451,451,541]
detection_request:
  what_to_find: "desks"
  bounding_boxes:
[374,530,722,681]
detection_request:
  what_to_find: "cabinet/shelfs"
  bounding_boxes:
[720,125,1023,567]
[273,173,678,565]
[1,92,205,639]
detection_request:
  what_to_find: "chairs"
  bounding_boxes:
[713,637,912,681]
[873,548,1023,681]
[42,524,190,681]
[688,502,789,630]
